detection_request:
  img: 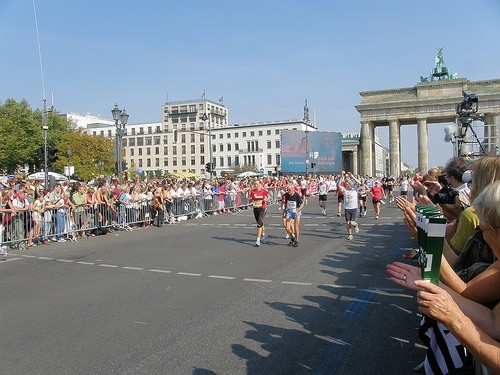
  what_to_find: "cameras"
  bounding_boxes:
[433,184,459,204]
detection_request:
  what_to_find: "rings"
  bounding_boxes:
[403,275,407,281]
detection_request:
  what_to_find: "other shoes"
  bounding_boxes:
[10,231,92,251]
[321,210,327,216]
[354,224,360,235]
[359,210,368,218]
[260,235,266,242]
[337,212,341,217]
[347,234,354,241]
[255,240,261,247]
[288,238,300,248]
[375,215,379,219]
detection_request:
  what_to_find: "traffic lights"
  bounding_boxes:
[206,162,211,173]
[48,147,58,162]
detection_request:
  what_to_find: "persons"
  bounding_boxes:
[370,182,386,219]
[316,178,329,214]
[337,171,361,240]
[0,156,500,375]
[248,181,271,247]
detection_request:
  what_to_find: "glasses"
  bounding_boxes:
[474,225,500,236]
[445,174,452,178]
[286,189,293,191]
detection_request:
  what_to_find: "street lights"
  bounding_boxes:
[112,103,129,172]
